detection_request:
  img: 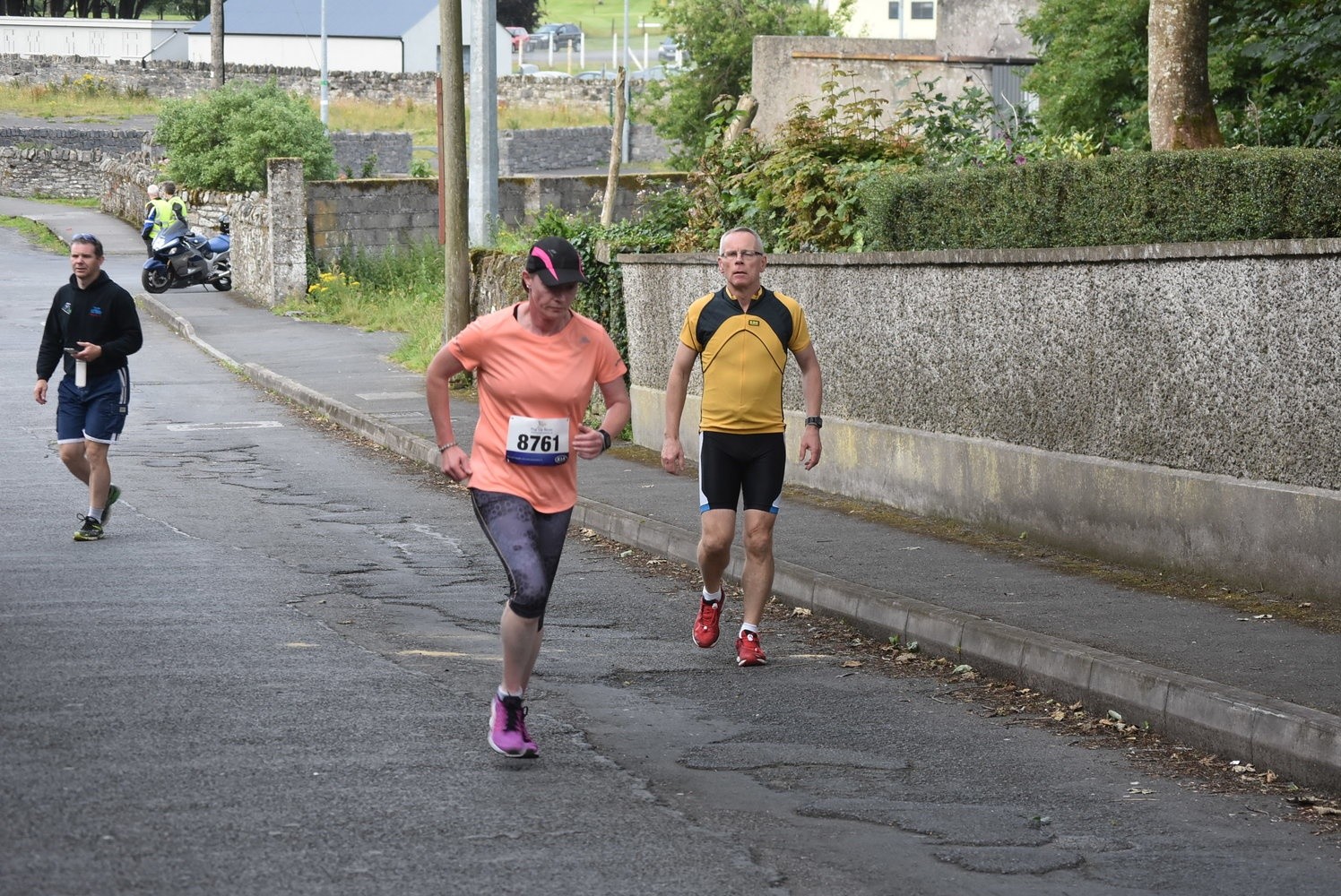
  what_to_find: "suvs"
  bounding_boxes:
[527,23,582,52]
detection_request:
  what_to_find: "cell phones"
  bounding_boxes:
[64,348,79,354]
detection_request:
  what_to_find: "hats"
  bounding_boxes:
[522,237,588,285]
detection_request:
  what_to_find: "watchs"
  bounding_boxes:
[805,416,823,429]
[596,429,611,454]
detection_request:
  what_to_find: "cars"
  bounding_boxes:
[518,63,570,81]
[658,36,678,62]
[504,26,530,53]
[631,65,690,83]
[577,70,617,79]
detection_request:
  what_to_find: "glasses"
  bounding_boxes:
[720,250,763,259]
[72,233,97,245]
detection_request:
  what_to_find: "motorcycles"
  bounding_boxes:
[142,203,232,294]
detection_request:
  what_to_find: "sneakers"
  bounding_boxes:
[735,629,767,667]
[102,485,121,527]
[488,694,526,756]
[74,513,104,540]
[693,586,726,647]
[521,706,539,756]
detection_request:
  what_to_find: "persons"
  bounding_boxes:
[34,233,142,542]
[425,236,631,758]
[142,181,187,259]
[660,226,825,665]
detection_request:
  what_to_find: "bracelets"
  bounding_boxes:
[439,441,459,452]
